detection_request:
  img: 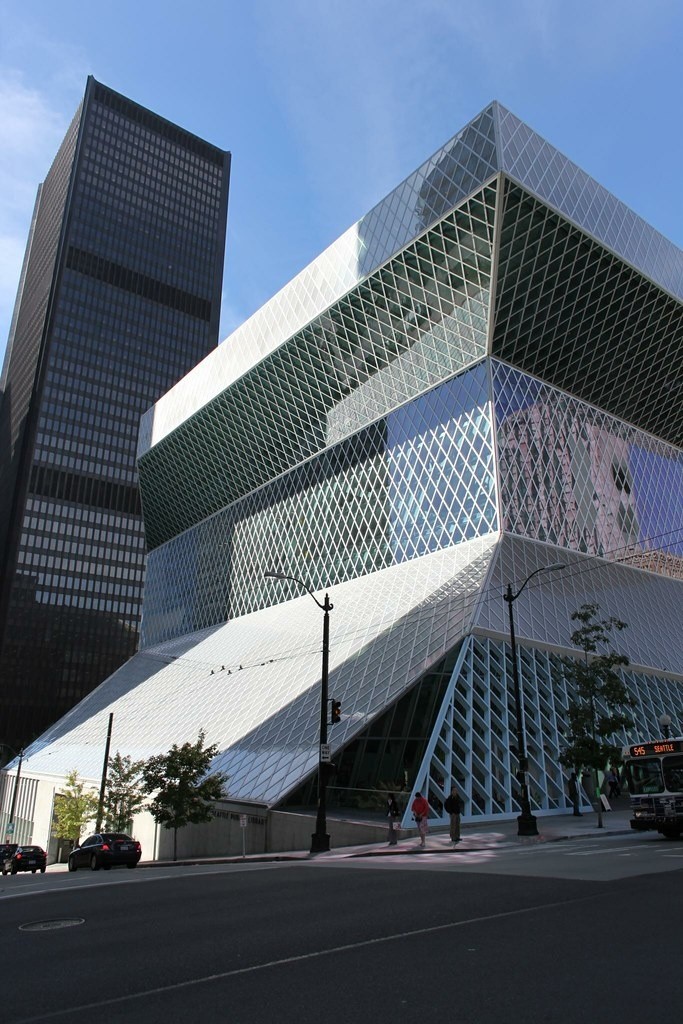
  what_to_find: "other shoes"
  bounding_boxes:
[573,812,583,816]
[451,840,458,843]
[456,838,462,841]
[388,842,396,846]
[417,844,425,847]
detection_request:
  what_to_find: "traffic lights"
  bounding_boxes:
[332,701,341,723]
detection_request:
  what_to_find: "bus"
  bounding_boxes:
[622,737,683,839]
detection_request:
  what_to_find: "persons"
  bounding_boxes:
[608,768,618,799]
[411,793,428,847]
[568,773,583,816]
[444,786,464,842]
[385,793,398,845]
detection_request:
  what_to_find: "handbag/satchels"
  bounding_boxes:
[414,817,422,822]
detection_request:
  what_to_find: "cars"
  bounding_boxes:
[68,833,141,872]
[0,844,18,872]
[3,846,47,875]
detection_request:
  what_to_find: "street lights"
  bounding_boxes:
[264,571,330,854]
[503,563,565,836]
[659,715,672,739]
